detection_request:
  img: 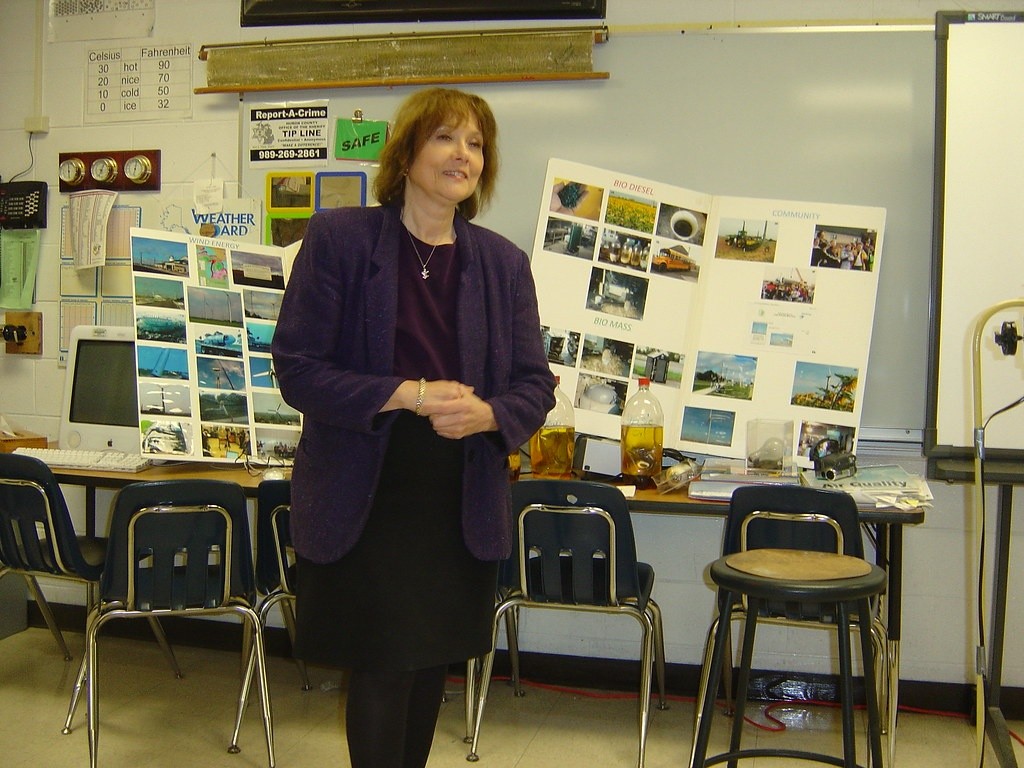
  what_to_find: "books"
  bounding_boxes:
[687,460,927,505]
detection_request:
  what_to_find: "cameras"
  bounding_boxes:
[814,452,857,480]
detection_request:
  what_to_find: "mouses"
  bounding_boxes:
[261,467,286,481]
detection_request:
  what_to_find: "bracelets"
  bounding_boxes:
[416,379,425,414]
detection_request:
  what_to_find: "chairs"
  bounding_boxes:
[465,478,667,768]
[0,454,183,735]
[89,478,278,768]
[690,484,887,768]
[229,481,313,755]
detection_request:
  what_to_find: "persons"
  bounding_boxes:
[762,277,813,303]
[272,89,556,767]
[258,438,298,459]
[201,427,253,458]
[810,231,874,273]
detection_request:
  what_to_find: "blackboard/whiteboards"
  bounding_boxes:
[920,10,1024,460]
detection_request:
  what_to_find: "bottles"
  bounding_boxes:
[505,447,521,484]
[620,377,664,491]
[528,376,577,481]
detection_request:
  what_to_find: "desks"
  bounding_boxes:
[0,441,925,768]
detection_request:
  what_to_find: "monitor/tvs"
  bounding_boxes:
[57,325,167,466]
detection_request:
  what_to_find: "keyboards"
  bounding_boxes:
[13,447,154,474]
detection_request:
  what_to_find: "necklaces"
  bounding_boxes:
[402,220,442,279]
